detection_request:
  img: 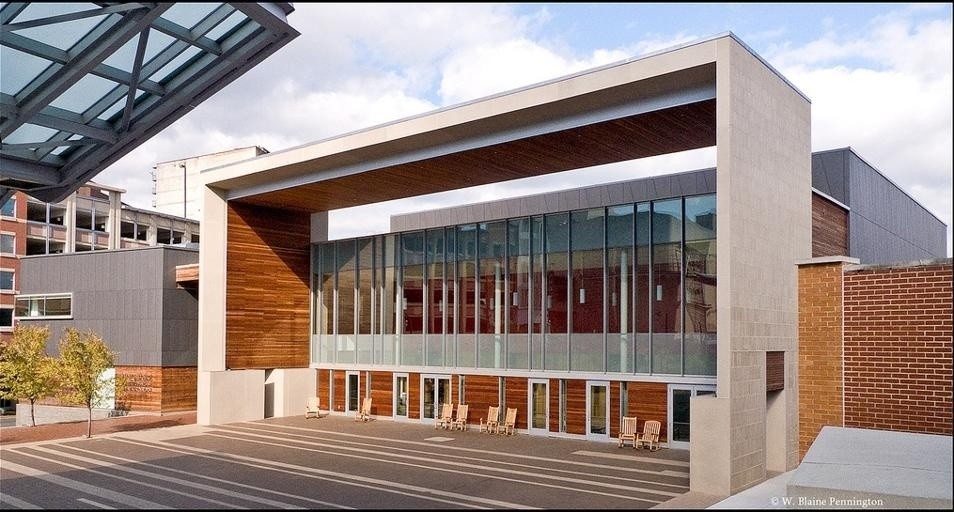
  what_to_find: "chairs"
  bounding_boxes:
[435,403,468,432]
[617,416,661,452]
[479,406,517,435]
[305,397,320,418]
[354,397,372,422]
